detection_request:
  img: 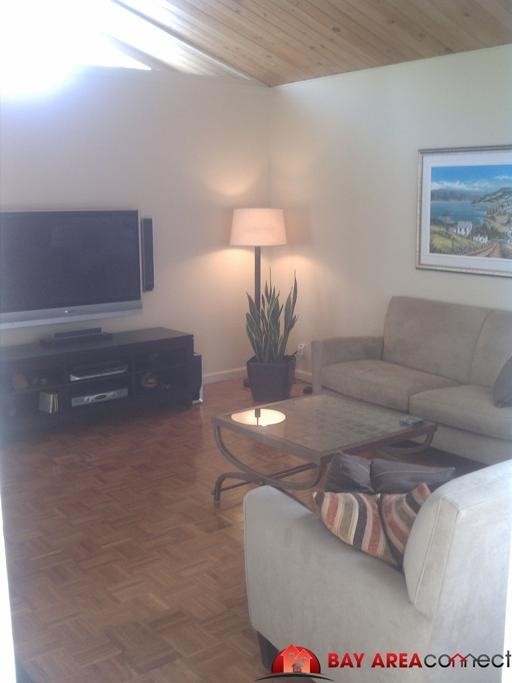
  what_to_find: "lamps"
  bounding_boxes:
[228,208,288,387]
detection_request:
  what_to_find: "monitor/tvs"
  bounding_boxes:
[0,211,145,331]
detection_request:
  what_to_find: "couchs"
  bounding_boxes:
[240,459,511,681]
[310,295,511,470]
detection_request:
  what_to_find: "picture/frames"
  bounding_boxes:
[414,144,512,278]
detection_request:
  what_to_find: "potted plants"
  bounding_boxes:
[245,270,299,402]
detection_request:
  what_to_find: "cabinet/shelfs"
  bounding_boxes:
[0,327,194,440]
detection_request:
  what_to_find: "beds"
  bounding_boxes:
[210,390,438,504]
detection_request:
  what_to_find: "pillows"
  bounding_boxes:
[312,481,432,568]
[494,356,511,407]
[323,450,456,495]
